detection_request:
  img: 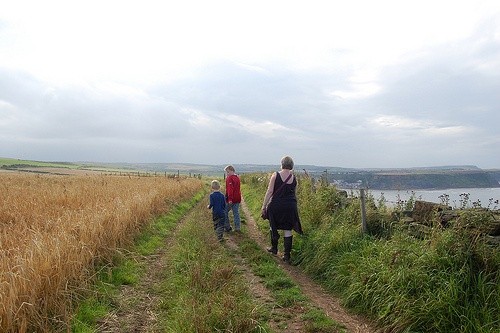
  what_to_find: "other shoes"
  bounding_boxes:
[234,229,241,232]
[224,228,231,232]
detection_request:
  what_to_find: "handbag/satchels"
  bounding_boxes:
[261,207,268,220]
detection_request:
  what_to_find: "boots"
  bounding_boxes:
[266,231,279,254]
[282,235,294,262]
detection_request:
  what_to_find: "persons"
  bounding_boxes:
[222,166,242,232]
[261,156,306,262]
[207,180,226,243]
